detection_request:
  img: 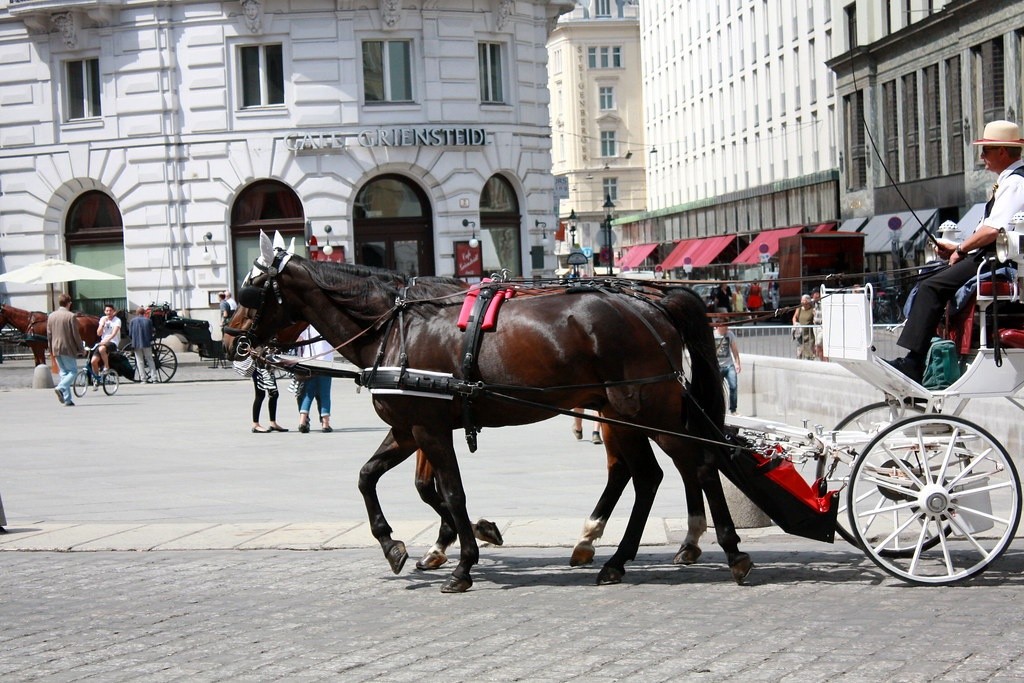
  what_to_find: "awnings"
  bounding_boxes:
[658,234,737,270]
[613,242,659,269]
[731,221,835,264]
[838,207,940,254]
[954,202,989,244]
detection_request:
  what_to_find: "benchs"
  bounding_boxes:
[183,318,232,369]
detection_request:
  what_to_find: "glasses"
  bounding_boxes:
[980,146,999,157]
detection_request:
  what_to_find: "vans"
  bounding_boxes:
[759,272,779,309]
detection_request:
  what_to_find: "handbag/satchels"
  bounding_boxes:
[49,352,61,374]
[233,355,255,377]
[922,337,962,393]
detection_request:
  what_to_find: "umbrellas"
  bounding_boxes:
[0,259,125,293]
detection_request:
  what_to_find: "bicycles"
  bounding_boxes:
[869,289,902,325]
[74,339,119,397]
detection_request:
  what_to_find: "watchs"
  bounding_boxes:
[954,243,967,258]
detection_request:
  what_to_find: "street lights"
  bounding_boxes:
[602,193,616,276]
[568,210,577,276]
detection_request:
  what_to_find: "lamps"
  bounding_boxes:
[626,150,632,159]
[535,219,548,245]
[322,225,333,256]
[463,219,478,248]
[586,173,593,179]
[604,163,609,170]
[203,232,212,260]
[650,145,657,153]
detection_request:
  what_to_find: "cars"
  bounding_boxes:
[691,284,745,314]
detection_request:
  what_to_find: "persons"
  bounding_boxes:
[250,339,290,433]
[0,495,8,532]
[879,120,1024,387]
[711,318,742,415]
[292,325,337,433]
[570,405,604,446]
[93,304,122,394]
[218,290,239,335]
[46,294,88,406]
[791,288,826,363]
[714,278,764,327]
[852,284,876,351]
[128,308,159,384]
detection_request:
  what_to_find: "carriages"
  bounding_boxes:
[221,225,1024,593]
[0,301,177,384]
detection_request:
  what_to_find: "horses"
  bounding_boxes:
[0,302,102,367]
[220,228,759,594]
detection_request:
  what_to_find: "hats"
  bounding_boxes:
[972,120,1024,147]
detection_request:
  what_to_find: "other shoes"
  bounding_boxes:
[572,421,583,439]
[879,355,921,383]
[153,380,160,384]
[252,428,271,434]
[305,414,310,430]
[93,382,99,391]
[593,434,603,444]
[55,387,65,404]
[298,424,309,433]
[65,401,75,407]
[323,425,333,432]
[101,367,110,375]
[270,425,288,432]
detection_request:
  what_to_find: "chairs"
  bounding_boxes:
[935,281,1020,354]
